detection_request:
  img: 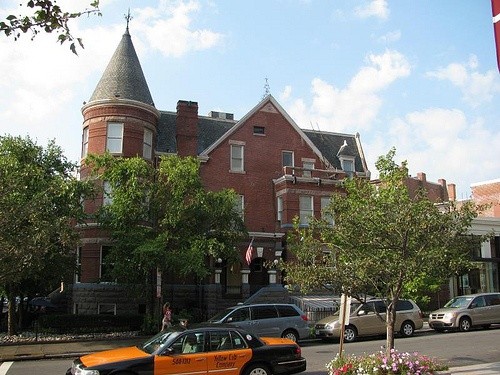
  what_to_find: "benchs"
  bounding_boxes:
[216,336,242,350]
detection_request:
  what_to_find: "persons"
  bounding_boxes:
[182,335,198,354]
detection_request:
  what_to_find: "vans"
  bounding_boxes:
[428,292,500,332]
[313,297,424,342]
[205,302,312,344]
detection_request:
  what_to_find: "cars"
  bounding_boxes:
[64,322,307,375]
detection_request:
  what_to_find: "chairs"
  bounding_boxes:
[181,334,199,354]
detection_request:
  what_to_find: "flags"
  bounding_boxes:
[245,241,253,266]
